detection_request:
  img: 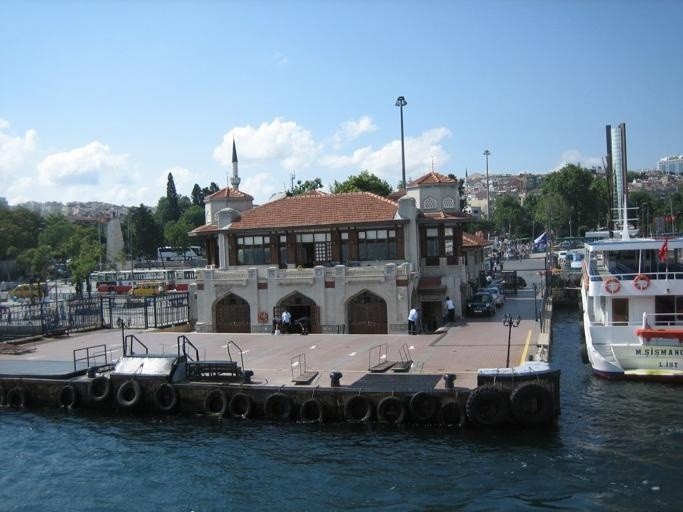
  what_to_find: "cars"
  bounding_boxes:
[467,271,527,317]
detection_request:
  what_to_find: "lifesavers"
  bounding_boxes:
[633,274,649,289]
[604,277,620,293]
[259,313,269,321]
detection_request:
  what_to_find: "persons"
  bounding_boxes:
[293,316,311,336]
[444,296,455,322]
[280,308,292,334]
[406,305,419,336]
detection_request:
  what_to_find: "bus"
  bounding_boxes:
[96,269,197,298]
[157,246,203,262]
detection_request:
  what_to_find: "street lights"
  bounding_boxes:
[395,96,408,188]
[483,150,491,240]
[503,313,521,368]
[532,282,541,322]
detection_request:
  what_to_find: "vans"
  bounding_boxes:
[10,283,49,303]
[558,251,568,262]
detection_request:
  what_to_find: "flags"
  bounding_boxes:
[658,239,670,264]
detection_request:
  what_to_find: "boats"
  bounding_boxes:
[579,193,683,379]
